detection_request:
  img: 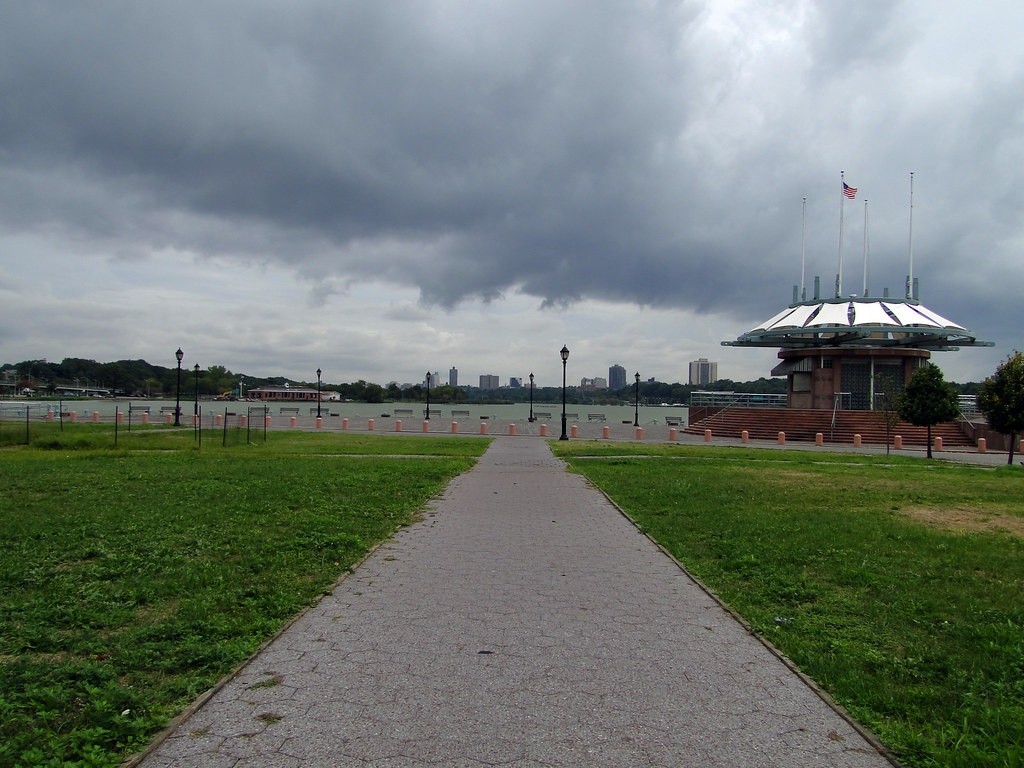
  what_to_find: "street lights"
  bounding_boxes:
[174,347,183,426]
[560,344,570,440]
[316,367,322,417]
[425,371,431,419]
[528,372,534,422]
[194,363,200,414]
[633,371,641,426]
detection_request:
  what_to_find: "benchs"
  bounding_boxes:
[52,405,68,412]
[280,408,299,416]
[127,406,151,415]
[560,413,579,422]
[422,409,441,418]
[665,417,685,426]
[249,406,270,416]
[587,414,606,422]
[393,409,413,418]
[310,408,329,416]
[159,407,182,415]
[451,410,469,417]
[534,412,551,421]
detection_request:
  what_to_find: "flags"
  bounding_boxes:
[843,182,857,200]
